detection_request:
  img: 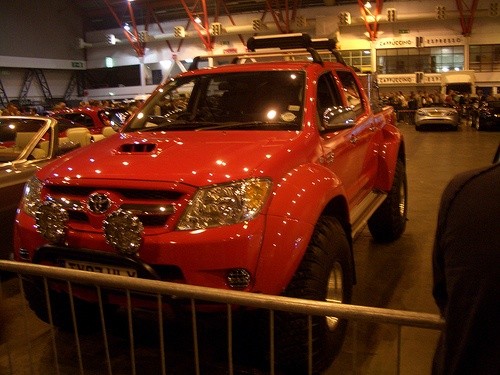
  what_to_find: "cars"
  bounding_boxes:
[0,105,175,262]
[466,99,500,130]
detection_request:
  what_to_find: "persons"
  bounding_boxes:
[0,97,217,125]
[430,142,500,325]
[376,85,500,127]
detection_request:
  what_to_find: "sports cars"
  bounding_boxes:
[414,102,459,130]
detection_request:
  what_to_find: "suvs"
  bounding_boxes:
[12,33,408,375]
